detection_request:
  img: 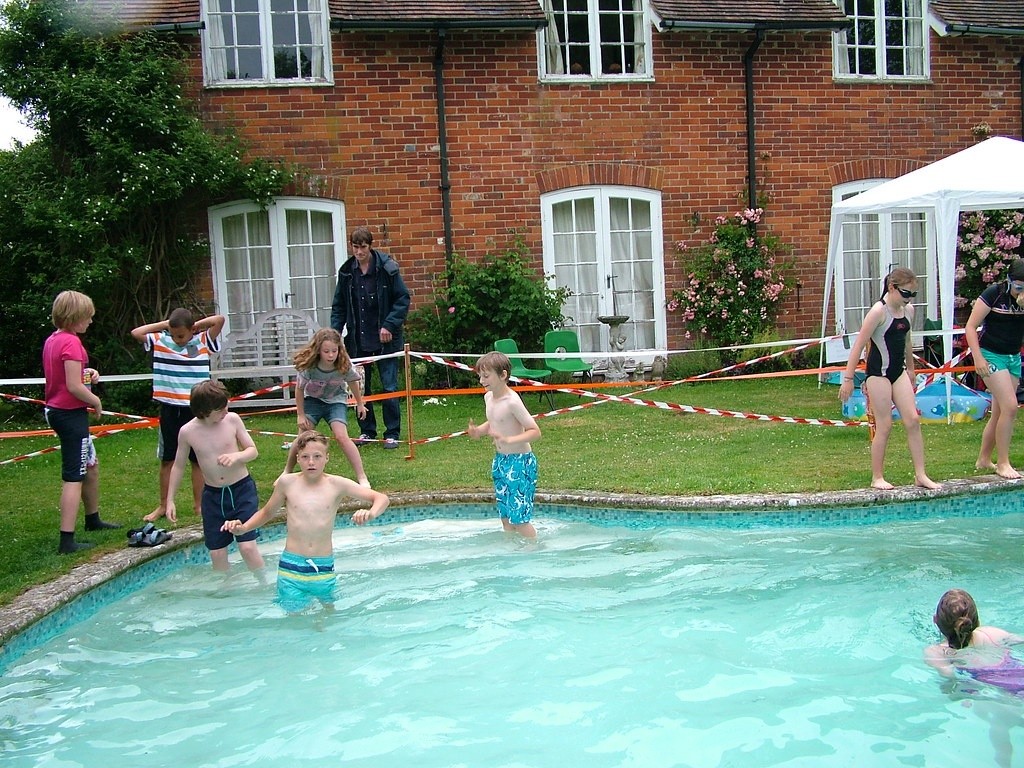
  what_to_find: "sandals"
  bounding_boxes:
[127,523,173,546]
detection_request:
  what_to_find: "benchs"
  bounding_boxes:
[206,304,322,407]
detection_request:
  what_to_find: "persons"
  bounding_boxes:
[330,227,411,449]
[131,308,225,522]
[838,267,942,489]
[273,327,371,489]
[220,429,390,600]
[965,258,1024,479]
[933,589,1010,649]
[165,378,264,572]
[468,352,541,536]
[609,333,627,375]
[42,291,123,556]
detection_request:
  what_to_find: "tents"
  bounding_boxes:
[817,136,1024,426]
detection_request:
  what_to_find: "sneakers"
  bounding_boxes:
[383,438,400,450]
[354,434,372,447]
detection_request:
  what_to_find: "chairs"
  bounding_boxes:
[924,319,976,386]
[539,331,598,404]
[493,338,554,412]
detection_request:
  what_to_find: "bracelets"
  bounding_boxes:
[844,376,853,383]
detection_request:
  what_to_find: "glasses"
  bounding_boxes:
[894,285,919,298]
[1006,277,1024,293]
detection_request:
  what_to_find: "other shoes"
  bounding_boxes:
[281,441,294,450]
